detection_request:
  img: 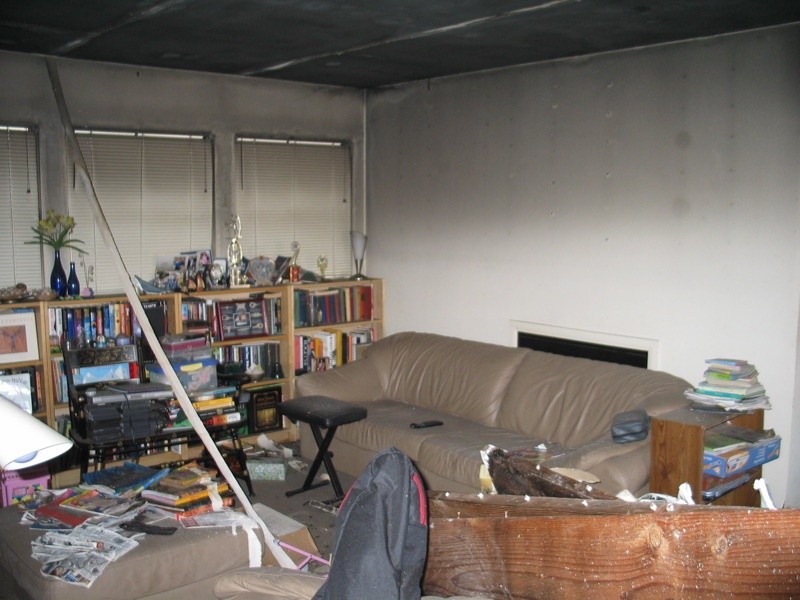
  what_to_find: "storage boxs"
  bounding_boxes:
[236,504,323,571]
[144,359,219,397]
[2,474,50,506]
[702,435,783,479]
[52,468,81,490]
[168,336,214,361]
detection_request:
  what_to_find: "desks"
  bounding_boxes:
[77,419,256,496]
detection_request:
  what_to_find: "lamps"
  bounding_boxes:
[0,395,74,472]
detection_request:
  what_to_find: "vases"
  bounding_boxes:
[68,263,82,298]
[50,249,67,298]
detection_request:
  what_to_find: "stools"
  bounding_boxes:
[279,396,367,507]
[1,492,262,600]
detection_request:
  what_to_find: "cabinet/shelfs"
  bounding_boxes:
[0,280,383,464]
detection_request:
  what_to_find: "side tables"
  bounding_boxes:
[652,406,766,508]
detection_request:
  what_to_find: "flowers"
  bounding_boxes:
[24,210,89,254]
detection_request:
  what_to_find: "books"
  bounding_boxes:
[182,286,375,386]
[49,301,170,403]
[154,389,251,427]
[683,355,771,411]
[16,461,235,532]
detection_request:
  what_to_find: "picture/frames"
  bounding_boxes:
[1,313,40,363]
[216,299,269,340]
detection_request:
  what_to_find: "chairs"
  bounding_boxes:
[215,446,429,600]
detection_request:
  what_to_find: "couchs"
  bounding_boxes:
[300,331,696,499]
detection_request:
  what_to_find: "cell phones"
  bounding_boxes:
[410,420,444,428]
